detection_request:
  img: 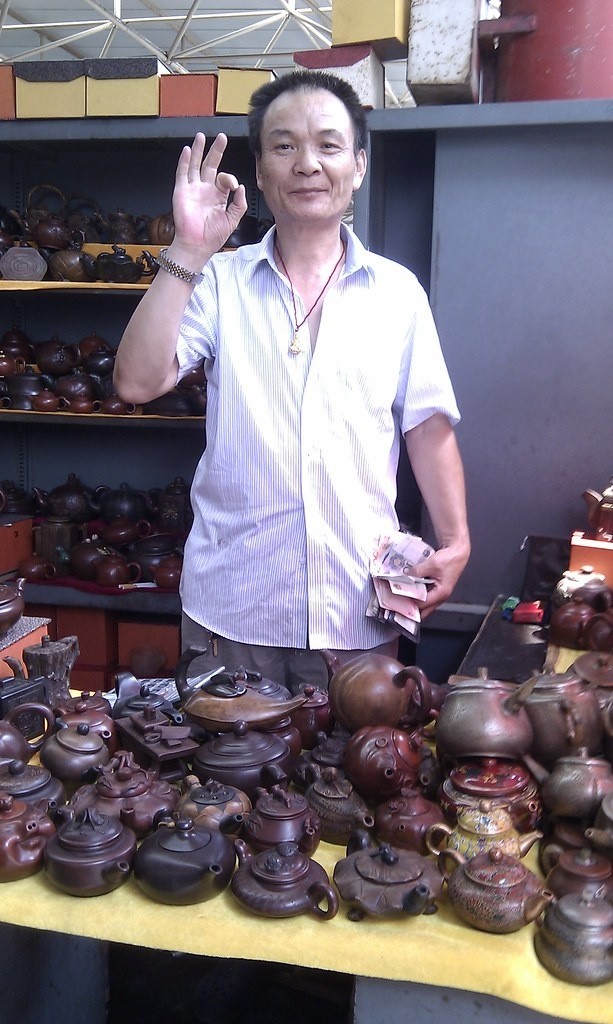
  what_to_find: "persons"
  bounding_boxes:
[113,70,470,696]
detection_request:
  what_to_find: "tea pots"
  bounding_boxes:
[0,182,613,986]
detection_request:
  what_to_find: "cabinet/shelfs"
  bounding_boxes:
[0,114,373,615]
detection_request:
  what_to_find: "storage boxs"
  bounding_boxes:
[406,0,481,106]
[0,61,16,120]
[159,73,218,117]
[20,603,181,694]
[568,531,612,591]
[215,64,278,115]
[293,45,385,111]
[331,0,409,60]
[13,60,85,118]
[84,56,159,117]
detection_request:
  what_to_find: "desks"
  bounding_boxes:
[458,594,550,686]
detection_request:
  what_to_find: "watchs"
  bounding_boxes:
[156,249,204,285]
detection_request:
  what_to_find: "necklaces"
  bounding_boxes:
[274,238,346,354]
